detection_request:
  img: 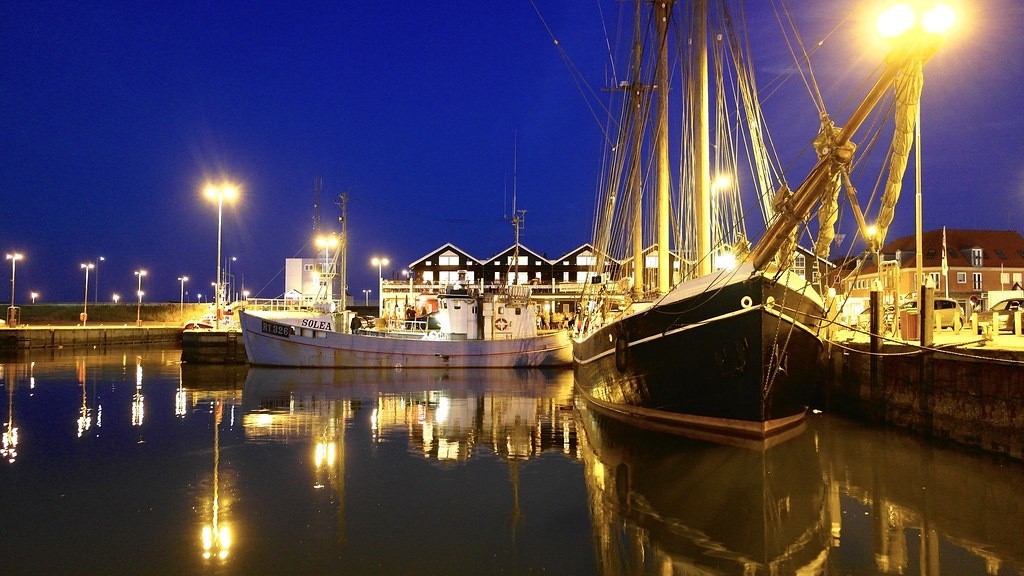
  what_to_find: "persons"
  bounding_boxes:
[350,314,362,334]
[422,307,428,315]
[406,305,416,329]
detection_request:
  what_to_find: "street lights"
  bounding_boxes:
[31,291,39,304]
[6,252,26,307]
[212,282,218,303]
[197,294,202,304]
[370,256,390,318]
[94,257,107,303]
[113,295,120,303]
[133,269,148,322]
[200,394,233,565]
[317,235,339,275]
[176,276,189,314]
[80,262,94,327]
[227,257,237,303]
[204,183,236,299]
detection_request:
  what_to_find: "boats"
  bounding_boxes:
[566,391,835,576]
[233,191,576,373]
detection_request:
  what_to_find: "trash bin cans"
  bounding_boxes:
[80,313,87,321]
[137,319,143,327]
[900,309,917,340]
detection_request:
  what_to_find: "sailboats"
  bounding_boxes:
[558,1,885,453]
